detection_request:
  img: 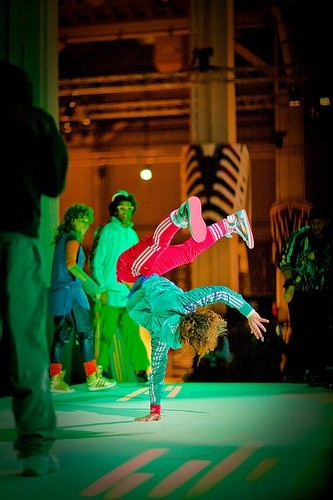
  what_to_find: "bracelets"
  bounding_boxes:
[149,404,161,414]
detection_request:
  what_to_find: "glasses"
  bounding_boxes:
[117,206,134,212]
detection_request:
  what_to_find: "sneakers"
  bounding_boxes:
[49,371,74,393]
[85,366,114,391]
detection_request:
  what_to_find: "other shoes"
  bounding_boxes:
[184,196,206,242]
[227,209,255,250]
[23,454,60,473]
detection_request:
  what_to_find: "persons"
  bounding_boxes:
[0,60,333,477]
[116,196,270,422]
